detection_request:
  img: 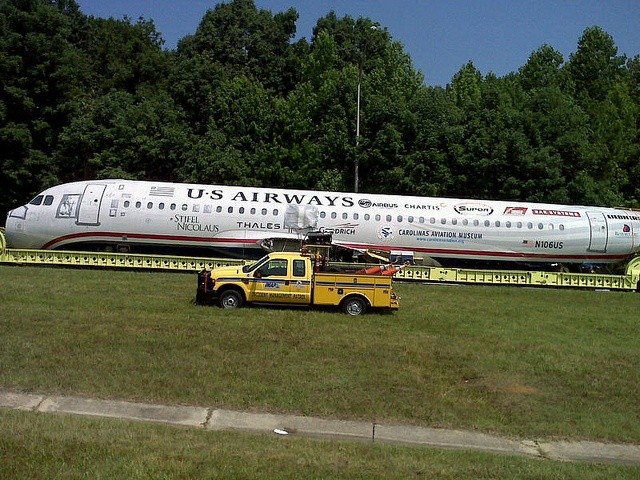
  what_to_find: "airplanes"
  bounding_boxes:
[1,173,640,274]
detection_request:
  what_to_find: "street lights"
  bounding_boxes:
[354,26,378,191]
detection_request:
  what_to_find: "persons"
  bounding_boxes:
[260,238,273,260]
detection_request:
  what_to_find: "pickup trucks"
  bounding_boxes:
[196,250,392,318]
